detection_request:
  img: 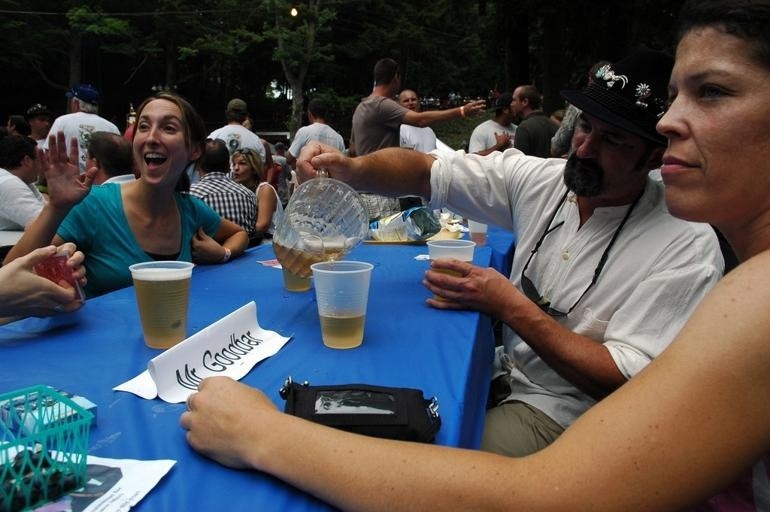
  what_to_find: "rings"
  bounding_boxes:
[55,302,67,312]
[187,392,200,413]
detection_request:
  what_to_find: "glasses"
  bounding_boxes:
[521,221,608,317]
[236,147,252,155]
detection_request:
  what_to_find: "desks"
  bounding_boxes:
[463,225,514,277]
[2,242,495,512]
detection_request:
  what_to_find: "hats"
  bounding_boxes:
[227,98,247,114]
[65,84,100,105]
[560,60,670,146]
[275,142,287,150]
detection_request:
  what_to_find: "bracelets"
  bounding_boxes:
[460,107,465,117]
[221,246,233,262]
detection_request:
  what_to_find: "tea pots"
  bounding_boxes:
[271,168,372,280]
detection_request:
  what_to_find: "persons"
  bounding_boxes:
[26,104,51,149]
[206,99,266,181]
[349,61,487,216]
[242,117,298,210]
[550,102,581,157]
[42,85,121,173]
[448,91,461,108]
[87,131,137,184]
[0,240,92,327]
[0,136,48,229]
[398,90,437,151]
[287,98,346,169]
[297,60,725,455]
[469,94,518,156]
[231,148,283,239]
[510,85,558,158]
[179,0,770,512]
[5,93,251,301]
[188,138,258,246]
[420,96,440,112]
[6,116,28,137]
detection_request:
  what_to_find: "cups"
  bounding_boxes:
[128,260,197,350]
[426,240,478,297]
[282,268,313,291]
[469,219,489,247]
[310,261,373,349]
